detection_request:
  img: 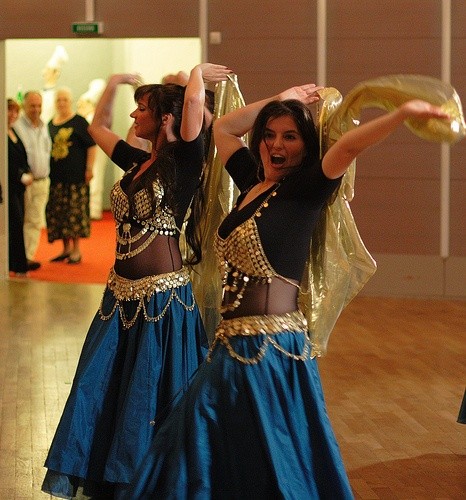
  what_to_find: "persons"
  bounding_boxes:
[7,62,250,500]
[122,83,454,500]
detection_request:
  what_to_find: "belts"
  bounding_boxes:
[28,177,49,181]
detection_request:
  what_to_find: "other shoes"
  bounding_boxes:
[9,262,40,274]
[51,254,80,264]
